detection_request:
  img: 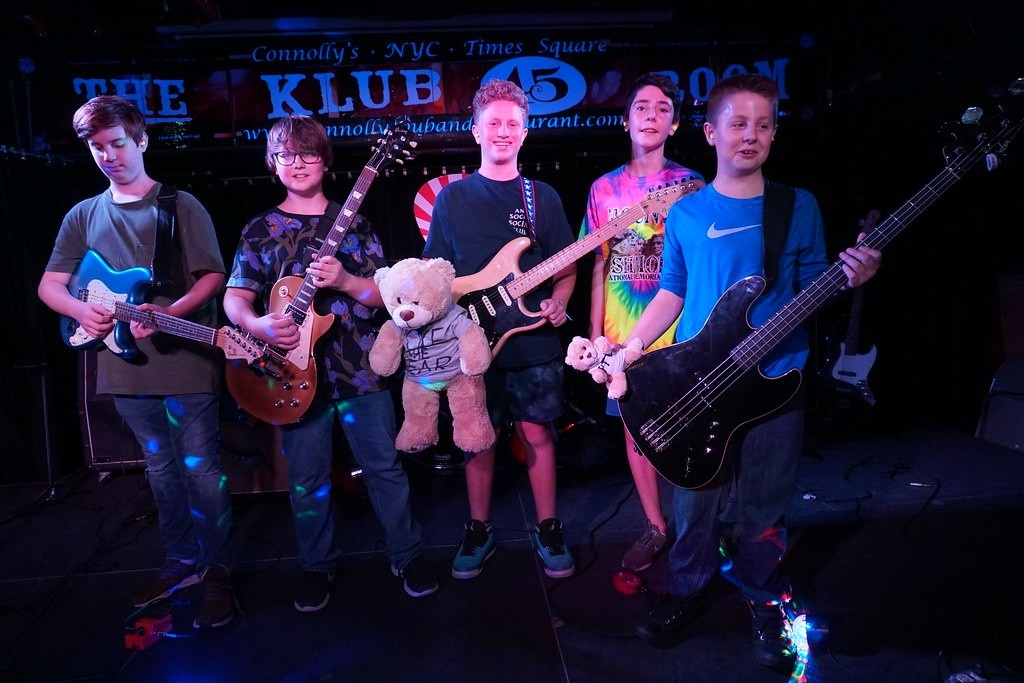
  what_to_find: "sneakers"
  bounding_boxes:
[747,600,798,665]
[391,552,440,597]
[134,571,202,609]
[534,517,575,577]
[622,524,668,571]
[636,591,705,642]
[293,570,331,612]
[451,519,495,579]
[192,564,241,627]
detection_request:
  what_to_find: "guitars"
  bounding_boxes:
[813,207,880,414]
[223,121,419,428]
[450,176,706,361]
[58,249,272,367]
[615,100,1023,492]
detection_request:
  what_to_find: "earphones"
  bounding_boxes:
[141,140,145,146]
[710,133,714,139]
[474,133,479,137]
[623,122,629,126]
[522,136,525,139]
[671,126,678,131]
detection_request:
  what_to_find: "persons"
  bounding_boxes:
[419,78,578,578]
[576,73,884,669]
[224,115,441,613]
[38,95,237,629]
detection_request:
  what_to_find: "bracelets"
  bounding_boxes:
[552,298,566,311]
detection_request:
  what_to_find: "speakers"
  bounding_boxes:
[81,347,292,496]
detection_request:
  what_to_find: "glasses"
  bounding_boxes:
[273,150,323,166]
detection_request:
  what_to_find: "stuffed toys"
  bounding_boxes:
[564,333,643,401]
[368,257,498,454]
